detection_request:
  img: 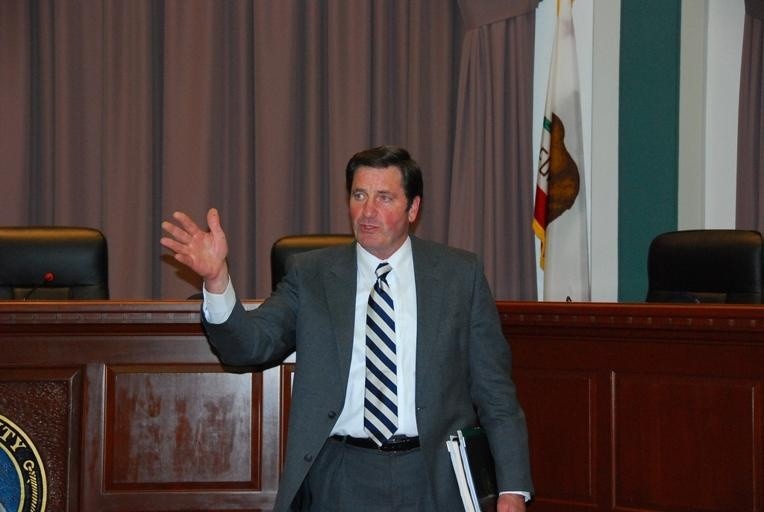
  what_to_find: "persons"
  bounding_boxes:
[160,144,534,512]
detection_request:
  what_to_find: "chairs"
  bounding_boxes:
[267,231,351,290]
[643,227,762,306]
[2,222,110,303]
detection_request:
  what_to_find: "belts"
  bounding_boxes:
[331,433,420,456]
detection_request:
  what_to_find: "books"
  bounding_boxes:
[446,425,498,512]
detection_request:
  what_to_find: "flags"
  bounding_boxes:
[534,0,593,305]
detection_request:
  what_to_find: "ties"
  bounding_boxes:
[364,261,398,448]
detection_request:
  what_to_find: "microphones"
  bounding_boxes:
[24,272,54,300]
